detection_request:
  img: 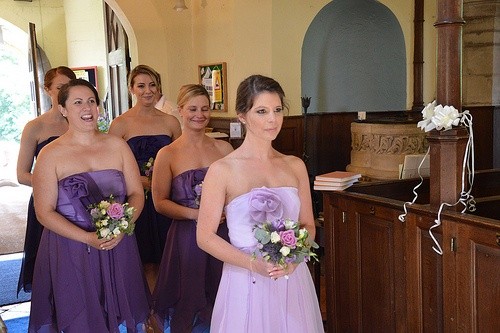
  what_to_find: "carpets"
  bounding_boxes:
[0,257,32,308]
[4,316,209,333]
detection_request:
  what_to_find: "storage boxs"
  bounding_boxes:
[205,126,214,133]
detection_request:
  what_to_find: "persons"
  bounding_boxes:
[27,78,148,333]
[196,74,325,333]
[108,64,182,263]
[17,66,77,299]
[152,84,234,333]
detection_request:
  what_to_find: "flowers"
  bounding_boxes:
[140,157,154,200]
[97,114,111,133]
[417,99,460,132]
[85,193,138,240]
[254,218,321,271]
[193,179,203,209]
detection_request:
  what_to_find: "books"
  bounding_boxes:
[314,169,361,192]
